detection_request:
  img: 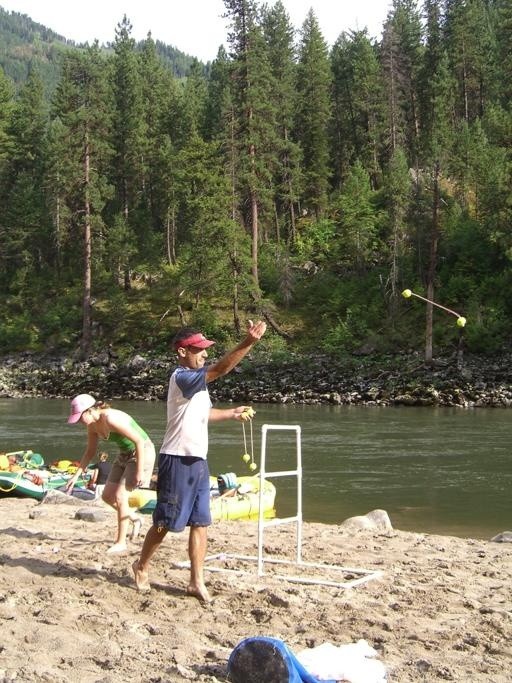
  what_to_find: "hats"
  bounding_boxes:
[173,332,215,349]
[67,394,96,425]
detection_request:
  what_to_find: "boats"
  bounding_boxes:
[0,447,276,522]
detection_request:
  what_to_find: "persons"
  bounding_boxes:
[131,317,269,603]
[64,391,156,554]
